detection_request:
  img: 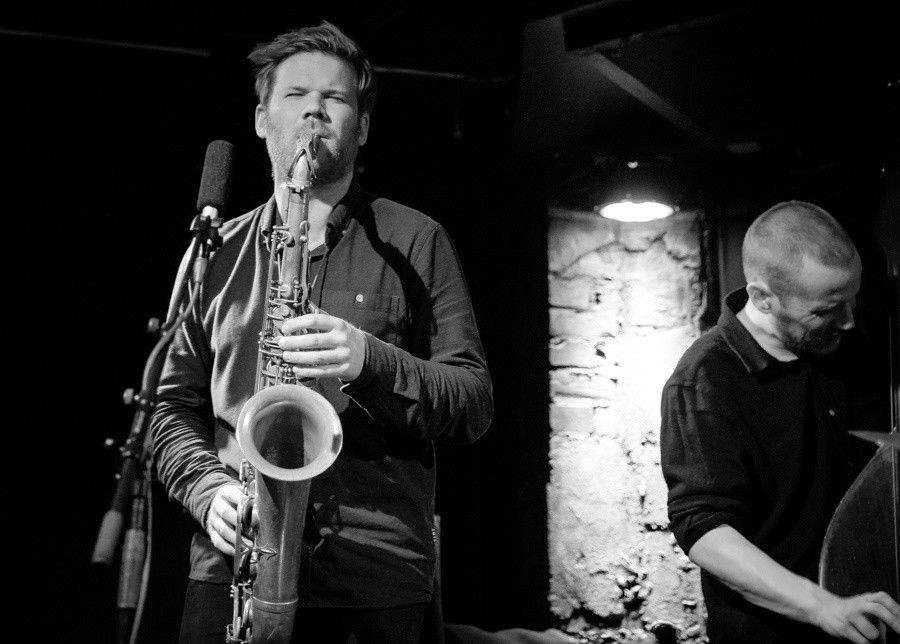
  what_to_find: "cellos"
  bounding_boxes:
[814,421,900,644]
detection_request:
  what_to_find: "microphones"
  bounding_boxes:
[193,139,237,284]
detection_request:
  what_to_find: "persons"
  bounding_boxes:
[141,22,494,643]
[660,200,899,644]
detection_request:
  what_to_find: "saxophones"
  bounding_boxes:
[223,132,345,644]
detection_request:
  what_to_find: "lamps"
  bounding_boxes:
[593,157,680,222]
